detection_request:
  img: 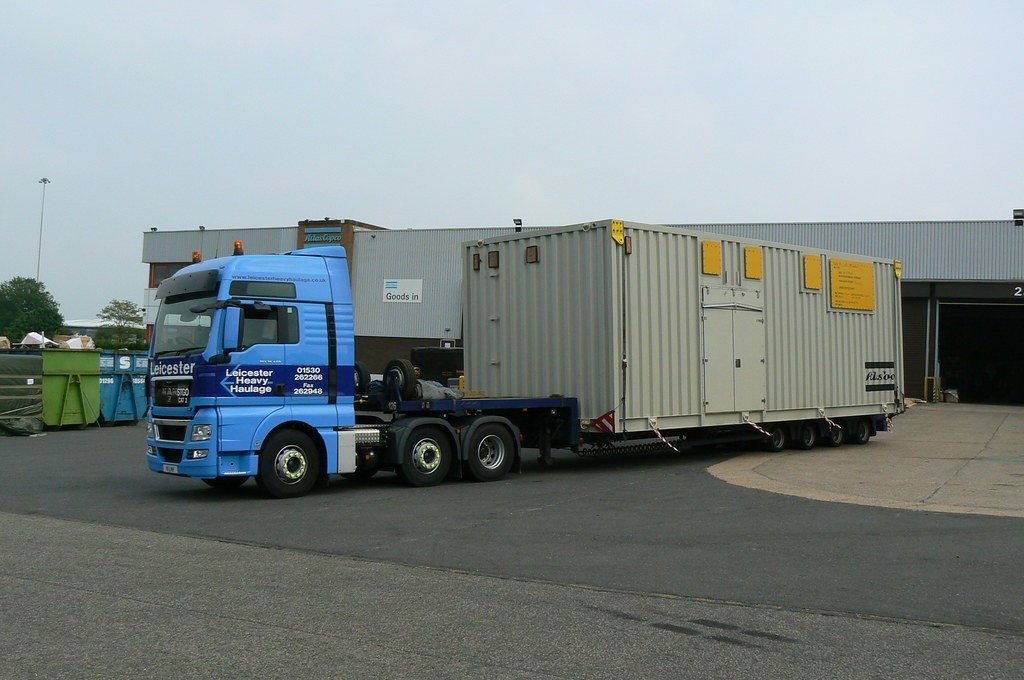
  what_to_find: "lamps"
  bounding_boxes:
[199,226,205,230]
[151,227,157,231]
[1013,209,1024,219]
[513,219,522,225]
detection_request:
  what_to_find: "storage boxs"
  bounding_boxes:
[943,388,959,402]
[0,349,43,436]
[0,331,94,349]
[460,218,904,434]
[43,349,102,430]
[97,348,150,422]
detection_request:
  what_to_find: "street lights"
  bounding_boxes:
[37,177,50,279]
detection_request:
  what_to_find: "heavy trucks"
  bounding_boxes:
[140,214,905,507]
